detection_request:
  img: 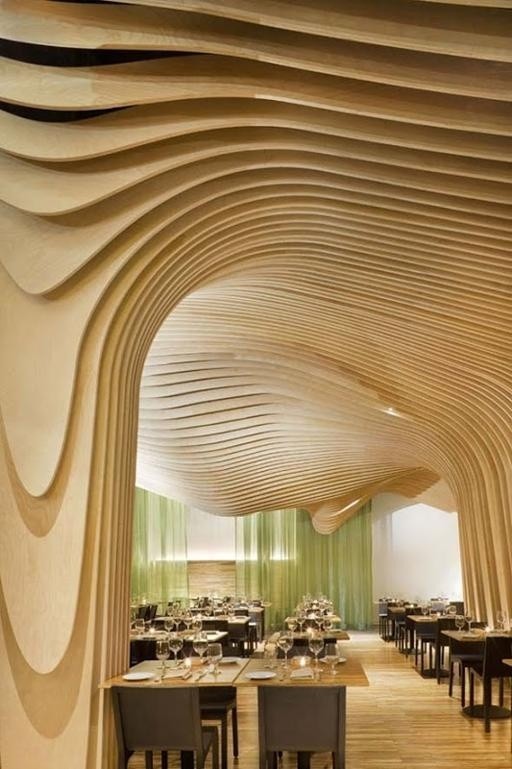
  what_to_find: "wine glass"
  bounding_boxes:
[454,609,507,639]
[168,632,183,670]
[277,630,294,670]
[308,631,325,672]
[156,640,171,669]
[288,592,334,637]
[134,615,218,641]
[207,643,223,674]
[420,600,457,619]
[166,589,264,621]
[192,630,209,673]
[324,642,340,675]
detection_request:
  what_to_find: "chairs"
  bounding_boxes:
[113,596,345,769]
[378,598,512,732]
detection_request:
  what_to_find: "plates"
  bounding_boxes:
[217,656,239,666]
[318,656,347,664]
[245,671,277,679]
[122,672,154,681]
